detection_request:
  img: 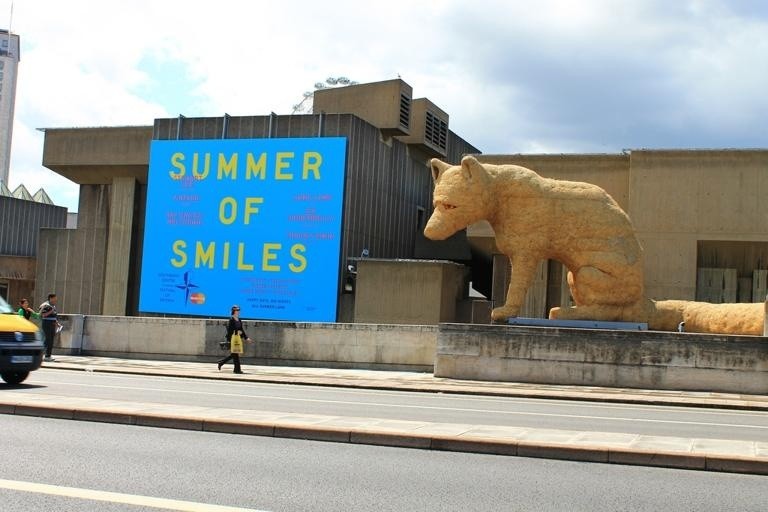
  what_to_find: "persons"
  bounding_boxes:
[216,305,256,375]
[37,292,63,364]
[17,297,42,323]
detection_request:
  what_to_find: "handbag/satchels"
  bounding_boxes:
[230,333,244,354]
[219,340,231,353]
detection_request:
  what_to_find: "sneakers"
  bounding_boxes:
[218,360,222,370]
[233,370,244,374]
[44,357,55,362]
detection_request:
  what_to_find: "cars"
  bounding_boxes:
[0,290,47,385]
